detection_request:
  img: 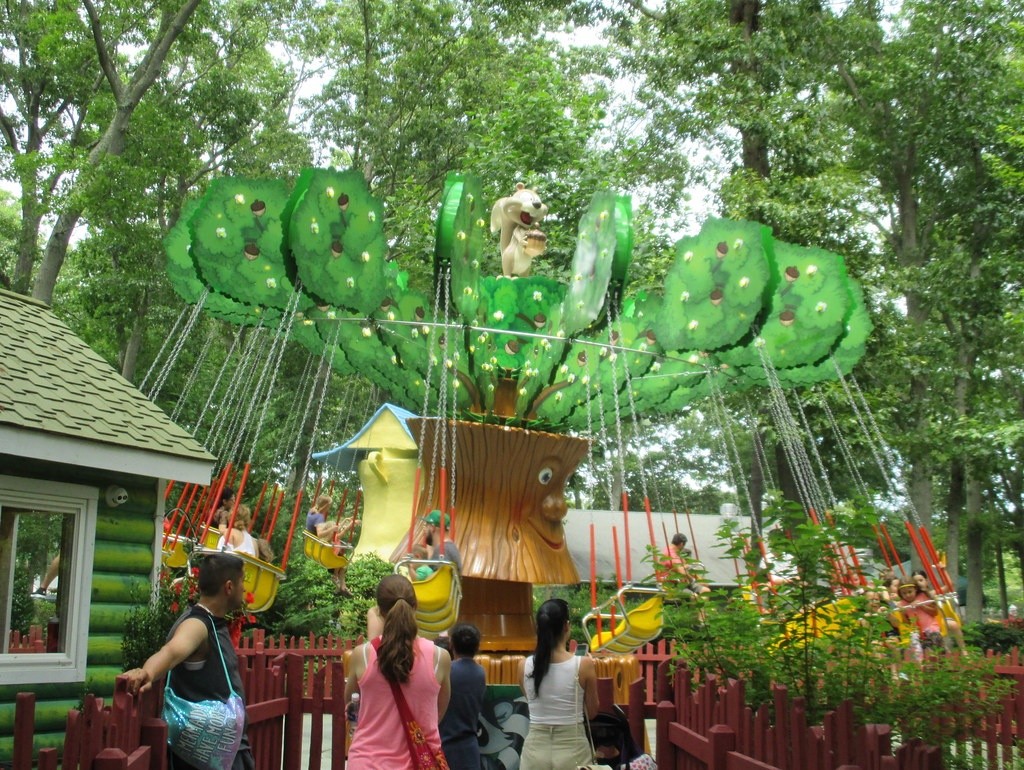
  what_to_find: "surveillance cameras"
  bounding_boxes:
[105,485,128,507]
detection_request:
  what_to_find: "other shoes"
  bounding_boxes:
[340,588,354,600]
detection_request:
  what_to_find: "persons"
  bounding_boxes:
[117,555,255,770]
[439,623,489,770]
[306,494,361,586]
[404,511,463,584]
[759,539,802,606]
[522,599,600,770]
[846,569,973,684]
[207,490,274,563]
[659,533,709,622]
[341,573,450,770]
[35,554,61,620]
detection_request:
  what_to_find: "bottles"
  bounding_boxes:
[47,613,59,653]
[348,693,361,740]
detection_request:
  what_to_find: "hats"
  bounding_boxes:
[421,511,450,530]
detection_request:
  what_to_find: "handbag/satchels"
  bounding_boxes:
[161,686,245,770]
[577,763,612,770]
[619,733,659,770]
[403,724,449,770]
